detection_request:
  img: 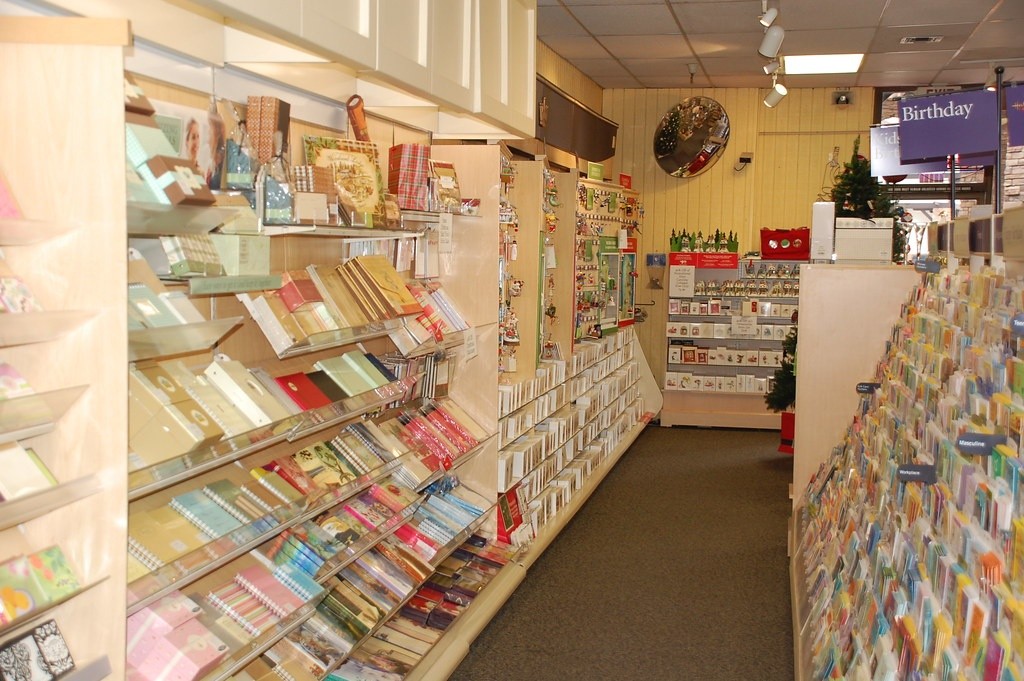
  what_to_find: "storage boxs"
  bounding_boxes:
[755,378,767,393]
[128,399,224,465]
[128,365,193,441]
[128,618,230,681]
[761,229,809,261]
[125,123,179,169]
[736,373,745,392]
[664,371,680,390]
[810,202,835,259]
[766,375,777,393]
[137,154,216,211]
[124,70,156,116]
[835,218,893,266]
[725,376,737,392]
[704,375,716,391]
[668,298,798,318]
[668,345,783,367]
[665,321,798,340]
[126,590,203,668]
[692,374,706,391]
[716,375,726,392]
[124,110,160,129]
[678,371,694,390]
[745,373,755,392]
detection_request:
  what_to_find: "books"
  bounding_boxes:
[1,109,1023,681]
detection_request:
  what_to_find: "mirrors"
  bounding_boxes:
[653,96,730,178]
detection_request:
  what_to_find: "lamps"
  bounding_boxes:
[763,62,780,75]
[760,7,778,27]
[758,26,785,59]
[764,84,787,107]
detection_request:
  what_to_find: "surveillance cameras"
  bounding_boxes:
[739,153,753,163]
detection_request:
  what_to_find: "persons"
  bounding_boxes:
[184,118,205,178]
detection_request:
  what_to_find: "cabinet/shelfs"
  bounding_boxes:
[0,18,664,681]
[661,250,809,430]
[788,208,1024,681]
